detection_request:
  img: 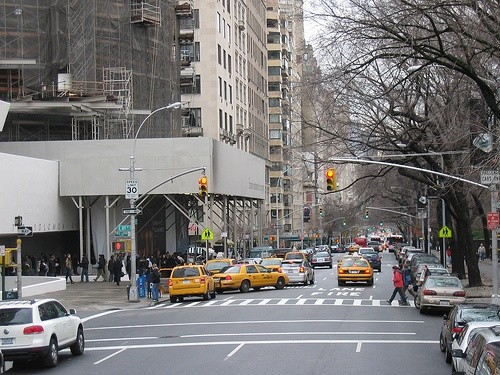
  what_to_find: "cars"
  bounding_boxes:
[239,233,450,291]
[280,259,314,286]
[336,258,375,286]
[311,252,334,268]
[414,275,467,314]
[438,302,500,375]
[212,264,289,294]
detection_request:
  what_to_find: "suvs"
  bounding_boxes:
[0,298,85,369]
[201,258,237,276]
[158,263,214,304]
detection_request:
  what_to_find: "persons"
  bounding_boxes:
[478,243,486,262]
[422,265,431,277]
[23,254,60,276]
[63,253,89,283]
[387,264,418,305]
[136,250,187,302]
[91,252,131,286]
[447,246,452,266]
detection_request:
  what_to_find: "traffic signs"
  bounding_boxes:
[122,207,144,215]
[17,226,34,237]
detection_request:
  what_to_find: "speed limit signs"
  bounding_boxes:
[125,179,139,198]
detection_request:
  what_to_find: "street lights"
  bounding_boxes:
[129,101,183,302]
[276,168,301,250]
[397,143,446,269]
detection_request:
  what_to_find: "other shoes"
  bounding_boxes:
[402,302,407,305]
[153,300,157,302]
[387,300,391,303]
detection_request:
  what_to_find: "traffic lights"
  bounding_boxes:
[326,168,337,190]
[198,177,201,197]
[4,247,18,266]
[114,241,126,252]
[200,176,208,197]
[365,210,368,219]
[343,220,347,228]
[319,208,324,217]
[379,220,382,226]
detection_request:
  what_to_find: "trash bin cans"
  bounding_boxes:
[138,276,145,297]
[127,285,130,300]
[3,290,17,299]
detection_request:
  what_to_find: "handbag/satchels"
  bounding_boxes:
[150,282,155,289]
